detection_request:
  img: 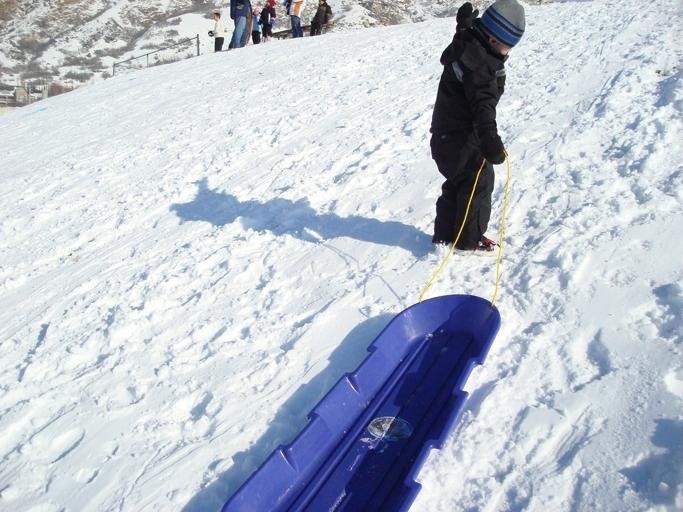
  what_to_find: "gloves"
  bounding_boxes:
[480,134,505,164]
[456,3,479,31]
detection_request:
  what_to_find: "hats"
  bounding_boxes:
[479,0,525,47]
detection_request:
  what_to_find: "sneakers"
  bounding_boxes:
[433,235,499,250]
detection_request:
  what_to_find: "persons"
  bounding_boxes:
[206,1,333,53]
[428,1,527,251]
